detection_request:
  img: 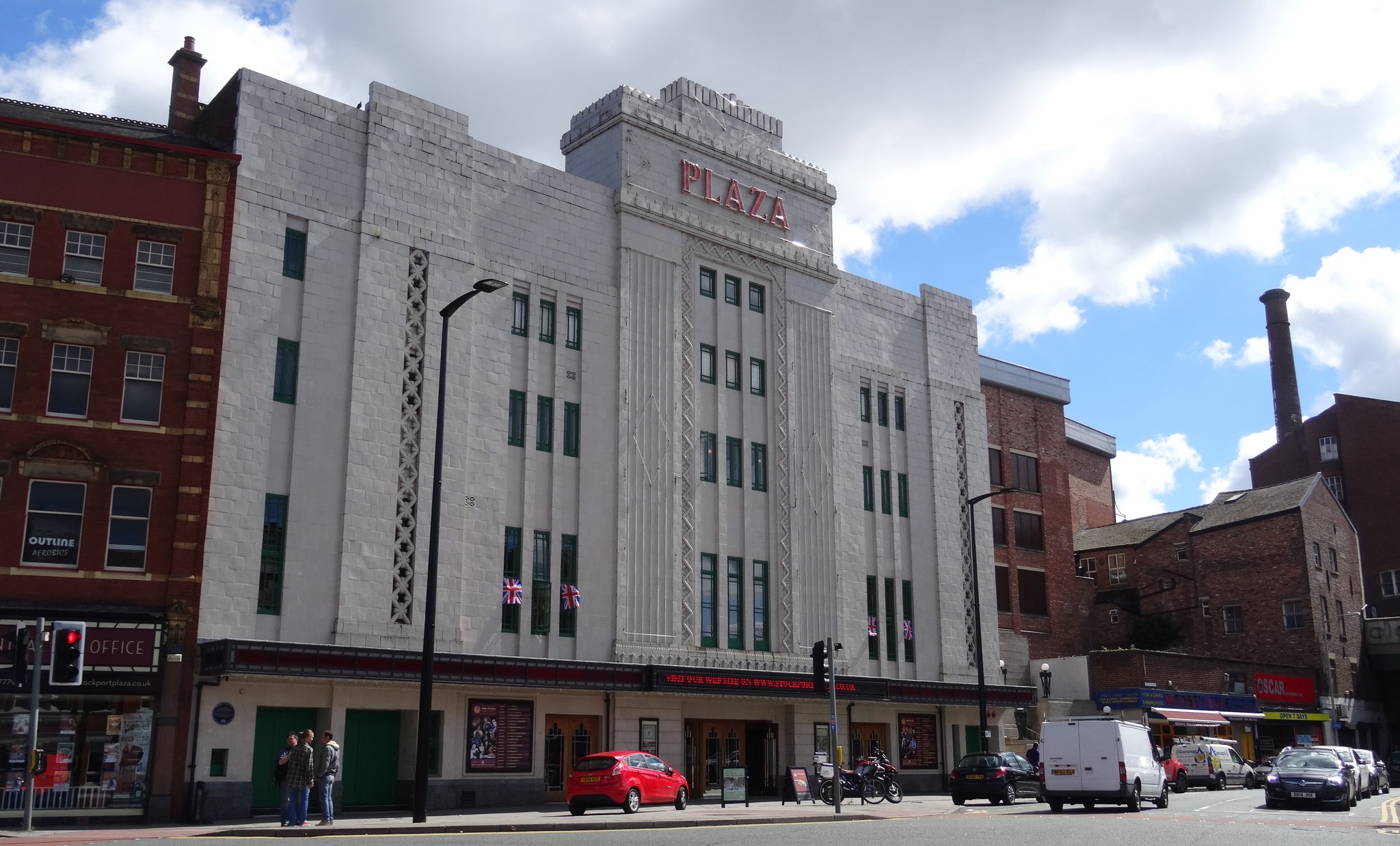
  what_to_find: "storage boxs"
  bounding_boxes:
[820,762,834,779]
[813,752,827,765]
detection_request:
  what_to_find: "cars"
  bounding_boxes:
[1351,748,1391,796]
[1252,756,1280,789]
[565,750,690,814]
[1149,742,1188,793]
[1264,746,1358,812]
[1307,745,1372,801]
[948,750,1047,806]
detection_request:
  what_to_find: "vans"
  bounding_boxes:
[1038,712,1169,813]
[1170,735,1256,790]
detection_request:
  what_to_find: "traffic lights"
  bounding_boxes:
[52,628,82,683]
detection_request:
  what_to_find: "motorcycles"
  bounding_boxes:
[812,741,903,804]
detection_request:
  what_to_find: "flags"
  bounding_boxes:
[868,616,877,637]
[502,578,522,604]
[903,620,913,640]
[560,584,580,610]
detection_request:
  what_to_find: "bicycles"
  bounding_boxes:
[818,762,887,806]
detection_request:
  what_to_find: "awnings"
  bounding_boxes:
[1151,707,1230,724]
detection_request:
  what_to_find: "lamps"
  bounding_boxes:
[1039,663,1053,699]
[1014,708,1029,740]
[999,659,1007,685]
[1101,706,1114,720]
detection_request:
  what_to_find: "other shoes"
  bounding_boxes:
[315,819,333,826]
[281,820,311,828]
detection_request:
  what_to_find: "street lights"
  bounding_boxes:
[411,278,508,824]
[1322,612,1361,746]
[966,485,1025,753]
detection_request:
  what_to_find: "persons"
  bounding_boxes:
[277,731,301,827]
[287,729,314,827]
[470,716,497,759]
[1025,742,1040,768]
[901,725,917,759]
[315,730,339,826]
